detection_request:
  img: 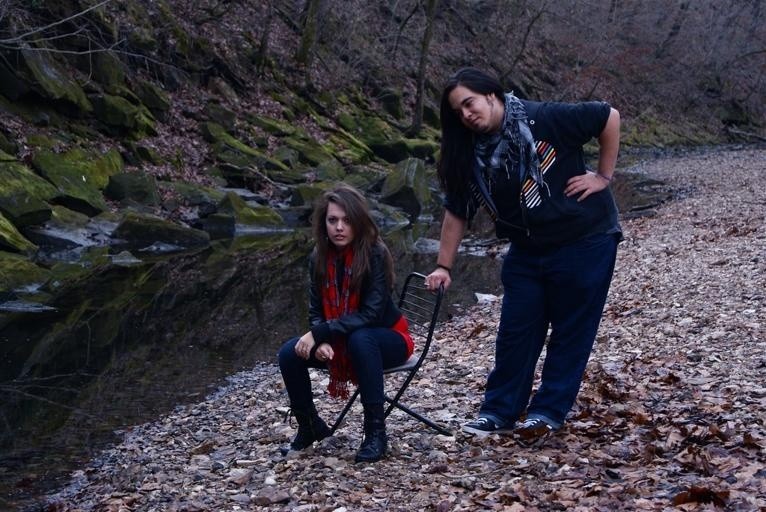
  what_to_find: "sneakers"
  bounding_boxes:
[514,416,558,444]
[459,415,515,436]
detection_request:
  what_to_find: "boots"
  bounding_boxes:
[353,419,389,462]
[288,416,333,449]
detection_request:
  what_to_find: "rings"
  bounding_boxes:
[321,355,326,359]
[424,281,430,286]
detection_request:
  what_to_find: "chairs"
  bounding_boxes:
[313,271,448,437]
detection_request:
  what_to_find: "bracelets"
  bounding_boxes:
[597,173,613,182]
[435,264,450,272]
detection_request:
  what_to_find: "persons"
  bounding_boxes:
[422,67,621,441]
[278,181,415,461]
